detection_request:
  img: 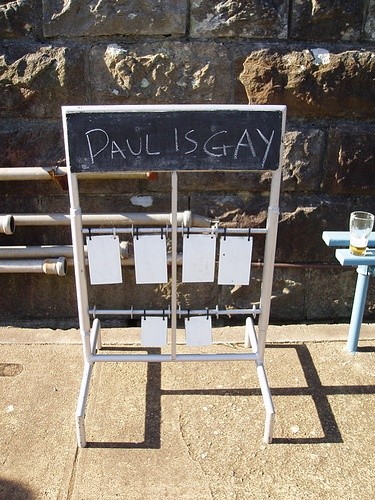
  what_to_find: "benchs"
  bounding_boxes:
[322,230,375,353]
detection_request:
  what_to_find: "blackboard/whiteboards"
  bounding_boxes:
[65,109,283,173]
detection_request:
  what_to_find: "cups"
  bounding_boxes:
[349,211,374,257]
